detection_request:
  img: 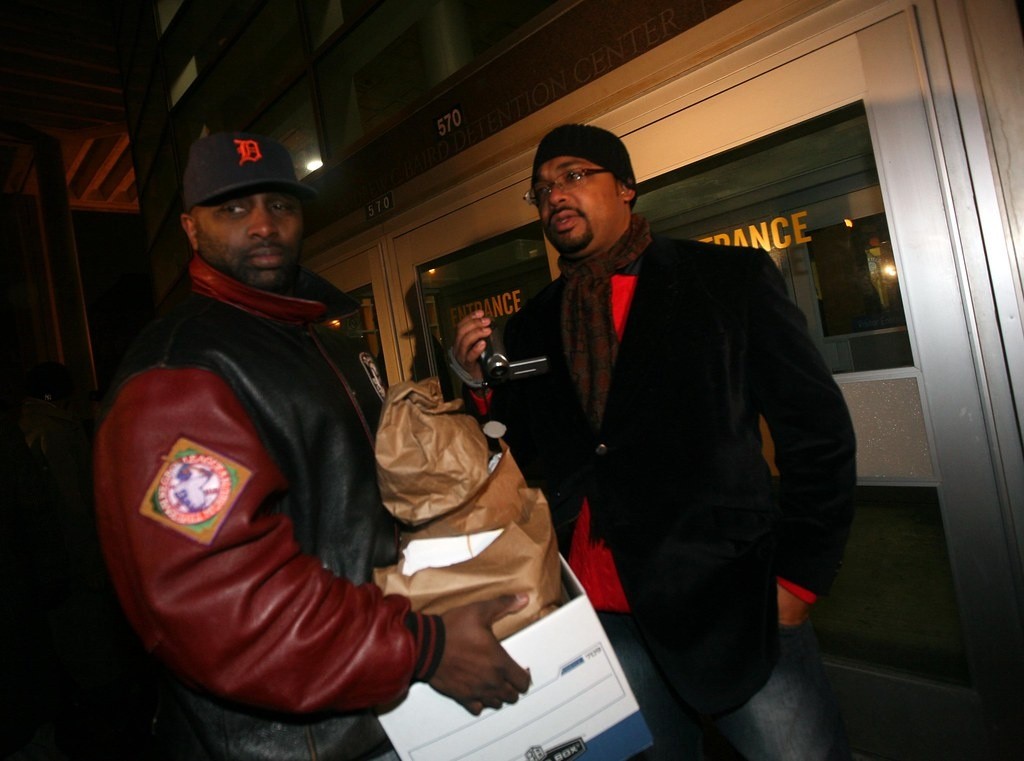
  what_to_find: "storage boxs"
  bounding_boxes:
[378,551,653,761]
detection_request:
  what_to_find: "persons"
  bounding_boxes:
[94,132,530,761]
[452,123,857,761]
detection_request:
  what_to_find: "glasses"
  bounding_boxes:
[522,167,610,206]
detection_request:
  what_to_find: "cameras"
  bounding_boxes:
[478,336,548,382]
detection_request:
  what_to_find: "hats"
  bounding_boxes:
[532,123,637,211]
[182,131,320,214]
